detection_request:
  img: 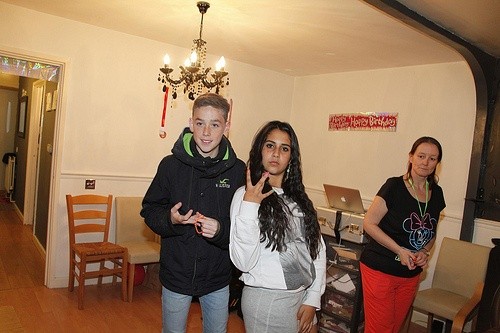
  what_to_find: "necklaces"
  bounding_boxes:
[407,172,429,224]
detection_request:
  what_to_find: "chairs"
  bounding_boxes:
[114,195,162,304]
[404,237,491,333]
[66,194,128,309]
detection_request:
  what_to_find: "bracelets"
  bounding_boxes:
[423,249,430,255]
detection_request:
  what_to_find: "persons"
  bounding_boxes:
[229,122,327,333]
[359,136,446,333]
[139,93,247,333]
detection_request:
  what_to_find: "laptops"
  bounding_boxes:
[323,183,367,216]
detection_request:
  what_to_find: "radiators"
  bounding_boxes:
[3,153,16,194]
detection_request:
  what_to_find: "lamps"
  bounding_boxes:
[159,1,233,107]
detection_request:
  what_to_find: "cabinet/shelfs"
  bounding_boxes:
[316,236,365,333]
